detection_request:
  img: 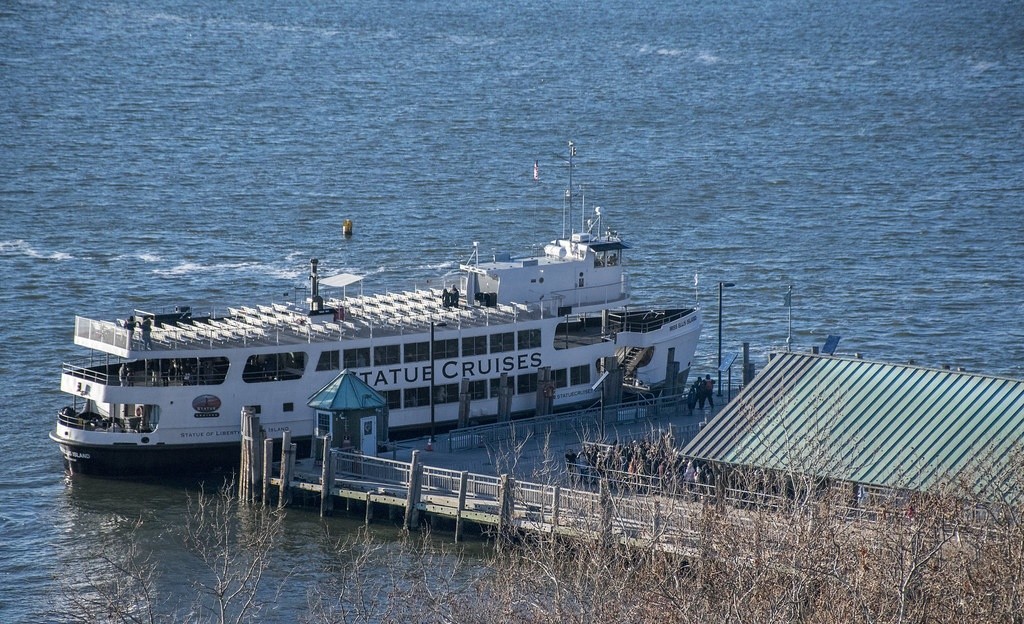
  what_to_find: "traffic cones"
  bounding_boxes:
[424,438,433,451]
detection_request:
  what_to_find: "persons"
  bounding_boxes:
[383,378,514,409]
[124,315,137,350]
[147,357,228,387]
[564,440,729,504]
[594,252,619,269]
[243,352,304,382]
[317,339,458,371]
[686,374,715,415]
[119,363,135,387]
[137,316,152,351]
[442,284,460,309]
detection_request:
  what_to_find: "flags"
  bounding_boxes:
[533,160,541,181]
[775,285,793,307]
[568,141,576,160]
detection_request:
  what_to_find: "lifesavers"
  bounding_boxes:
[544,384,556,398]
[134,406,143,418]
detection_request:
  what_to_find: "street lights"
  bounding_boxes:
[716,282,736,397]
[429,321,449,443]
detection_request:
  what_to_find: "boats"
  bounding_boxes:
[45,138,702,479]
